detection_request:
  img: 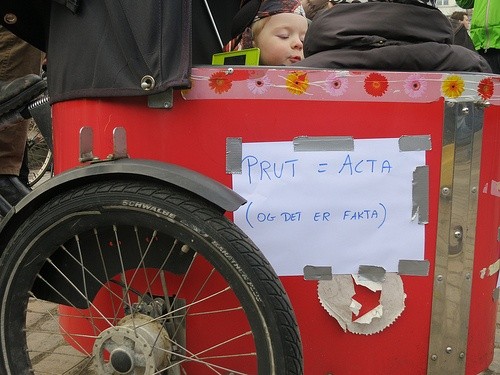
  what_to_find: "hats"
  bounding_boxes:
[230,0,261,37]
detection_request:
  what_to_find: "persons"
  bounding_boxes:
[451,10,475,49]
[289,0,494,73]
[301,0,335,19]
[240,0,309,67]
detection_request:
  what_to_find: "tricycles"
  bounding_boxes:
[0,42,500,375]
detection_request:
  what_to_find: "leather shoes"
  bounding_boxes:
[0,175,32,215]
